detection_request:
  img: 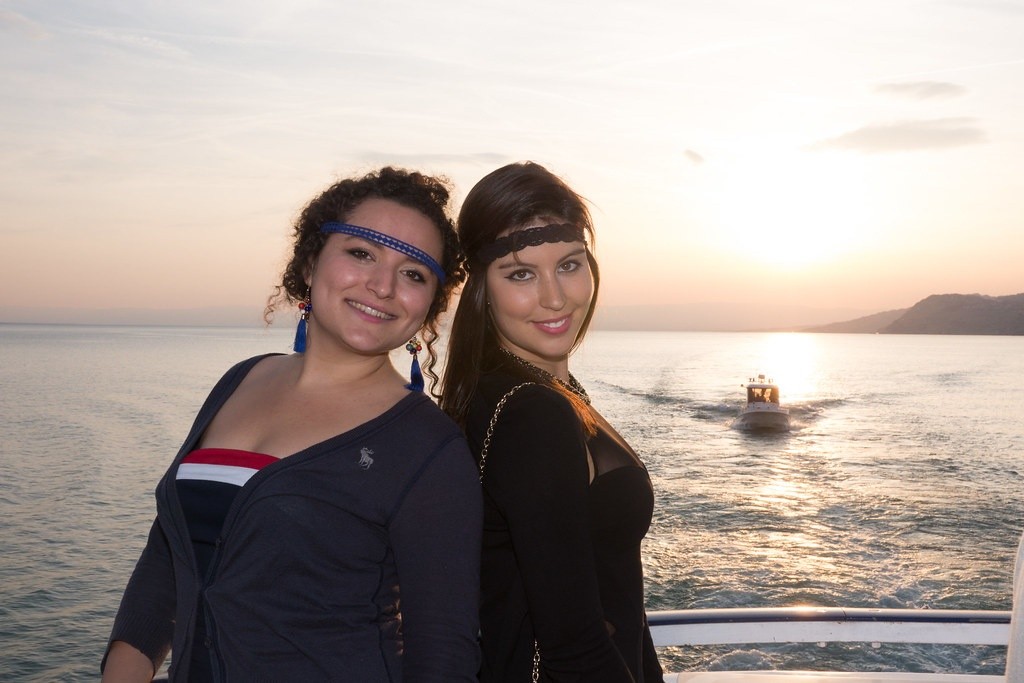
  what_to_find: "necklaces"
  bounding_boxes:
[497,346,591,405]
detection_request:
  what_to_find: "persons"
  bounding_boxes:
[100,167,484,683]
[440,162,665,683]
[754,393,766,402]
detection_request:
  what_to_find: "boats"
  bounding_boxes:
[729,372,792,433]
